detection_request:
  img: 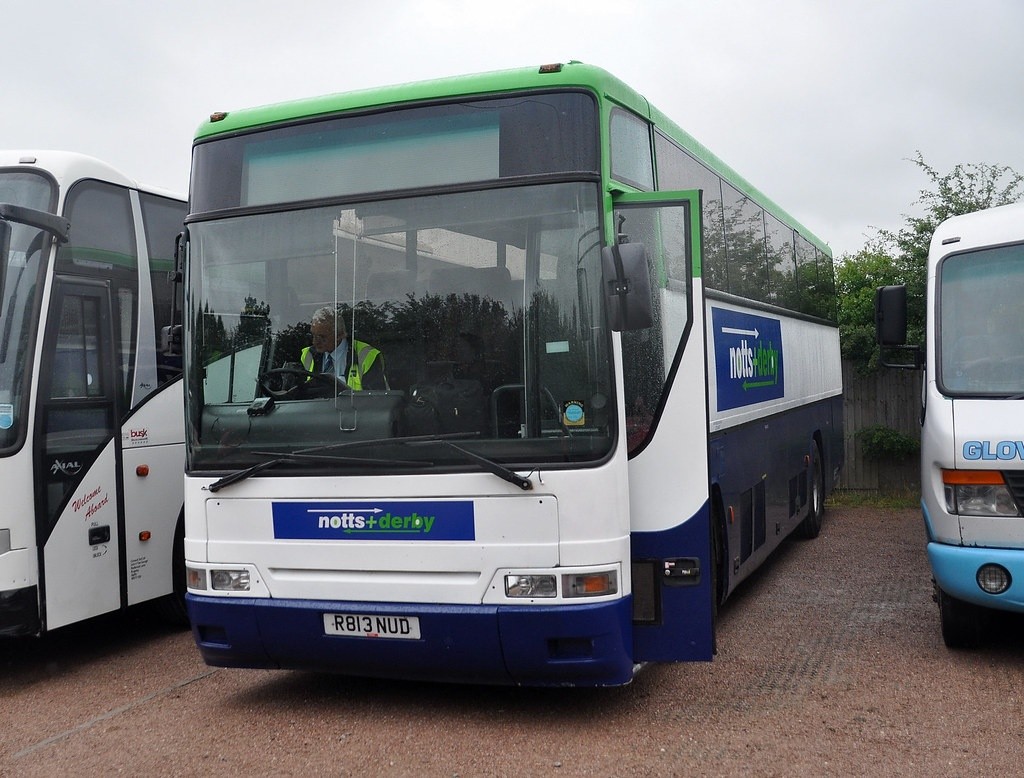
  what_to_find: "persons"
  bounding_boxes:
[301,308,384,390]
[452,332,496,392]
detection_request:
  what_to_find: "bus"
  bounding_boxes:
[873,193,1024,654]
[155,56,852,700]
[0,148,555,666]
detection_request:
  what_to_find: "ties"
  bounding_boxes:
[323,355,335,377]
